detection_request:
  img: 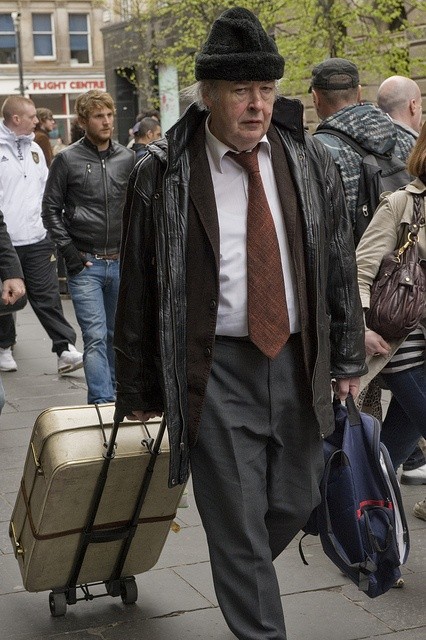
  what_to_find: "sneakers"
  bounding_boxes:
[0,346,18,372]
[413,498,426,520]
[401,464,426,485]
[58,343,84,374]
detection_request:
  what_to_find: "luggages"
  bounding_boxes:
[9,402,189,616]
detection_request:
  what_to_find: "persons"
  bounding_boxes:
[127,111,161,149]
[35,108,55,168]
[130,118,161,165]
[70,118,85,144]
[354,121,426,588]
[377,74,422,142]
[40,89,137,403]
[0,209,27,415]
[113,6,368,640]
[308,57,414,431]
[400,431,426,487]
[0,95,84,375]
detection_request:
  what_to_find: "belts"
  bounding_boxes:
[95,254,119,260]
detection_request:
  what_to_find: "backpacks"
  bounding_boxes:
[312,129,416,251]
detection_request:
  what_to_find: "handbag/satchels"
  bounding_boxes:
[365,244,426,339]
[299,392,409,598]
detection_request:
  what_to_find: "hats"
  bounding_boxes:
[196,6,284,81]
[308,58,359,93]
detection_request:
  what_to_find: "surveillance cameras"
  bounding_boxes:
[10,13,22,20]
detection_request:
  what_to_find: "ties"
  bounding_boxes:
[225,142,290,361]
